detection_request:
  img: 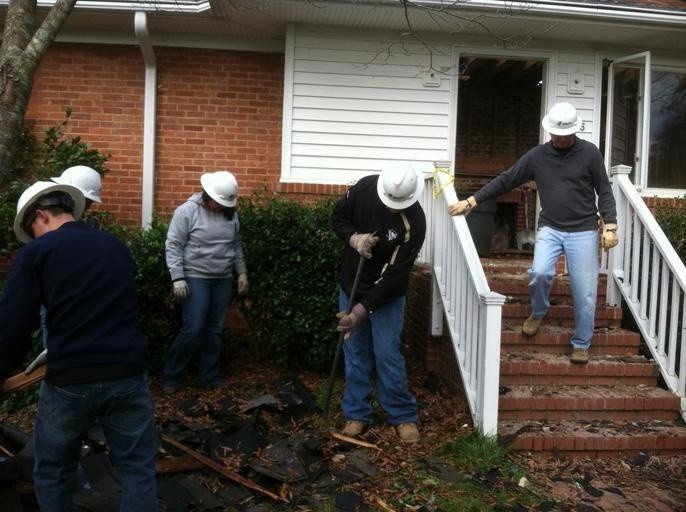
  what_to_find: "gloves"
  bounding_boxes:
[172,279,189,298]
[237,274,248,295]
[349,233,379,259]
[447,195,477,218]
[602,224,618,252]
[335,302,369,341]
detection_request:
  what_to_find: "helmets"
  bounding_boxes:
[13,180,86,245]
[199,170,237,207]
[541,102,582,135]
[49,165,102,205]
[375,160,424,209]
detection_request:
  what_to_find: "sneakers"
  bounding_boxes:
[341,420,367,437]
[521,306,550,336]
[570,348,588,363]
[396,423,420,444]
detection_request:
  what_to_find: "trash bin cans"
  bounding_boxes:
[457,192,498,258]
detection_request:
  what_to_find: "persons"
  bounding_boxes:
[450,101,619,364]
[0,181,164,512]
[40,164,104,349]
[332,162,427,446]
[163,170,250,394]
